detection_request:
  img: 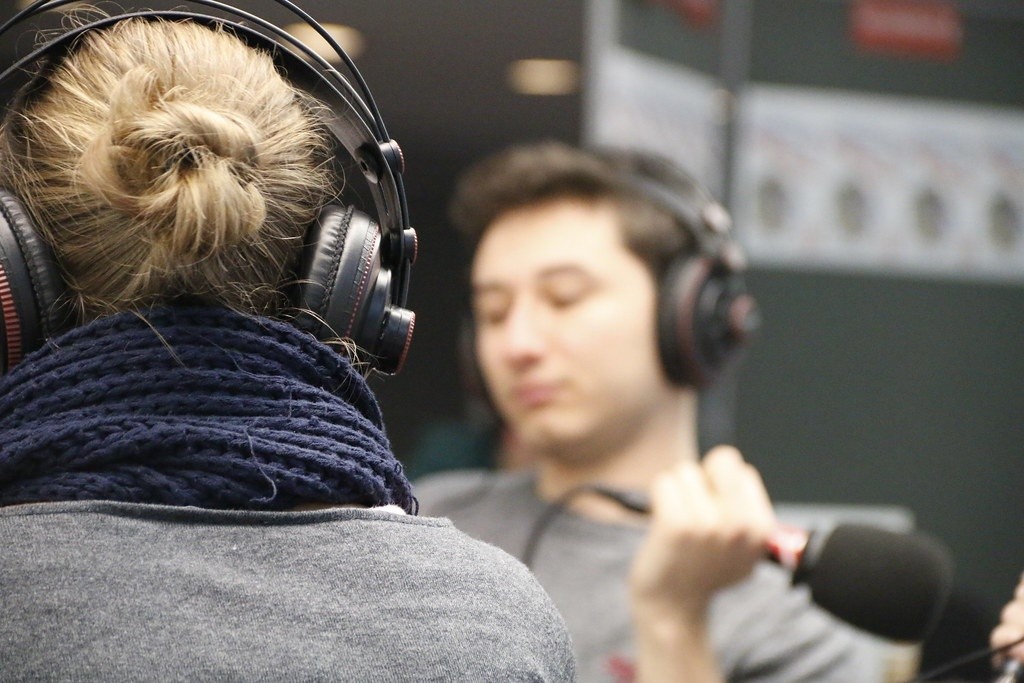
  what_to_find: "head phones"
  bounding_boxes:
[0,0,416,383]
[446,142,766,411]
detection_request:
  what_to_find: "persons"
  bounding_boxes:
[1,0,576,683]
[403,140,914,683]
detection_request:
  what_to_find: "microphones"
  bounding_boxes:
[613,482,955,647]
